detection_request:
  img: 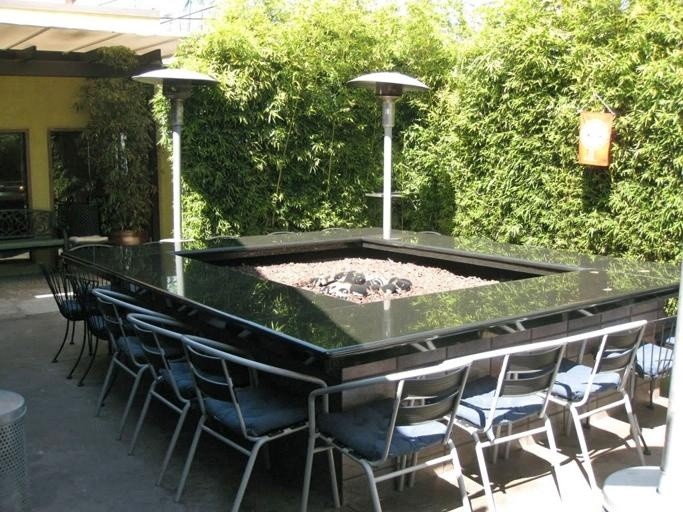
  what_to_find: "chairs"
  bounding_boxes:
[42,260,340,512]
[580,315,676,456]
[298,358,473,512]
[407,342,569,512]
[503,318,648,467]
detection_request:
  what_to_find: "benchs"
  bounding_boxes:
[0,209,70,275]
